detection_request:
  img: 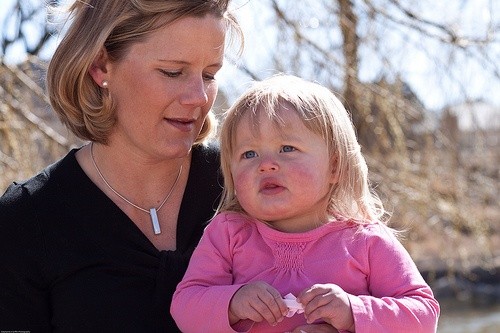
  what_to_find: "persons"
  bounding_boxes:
[0,0,240,333]
[168,74,441,333]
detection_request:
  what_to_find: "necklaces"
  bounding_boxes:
[89,139,186,235]
